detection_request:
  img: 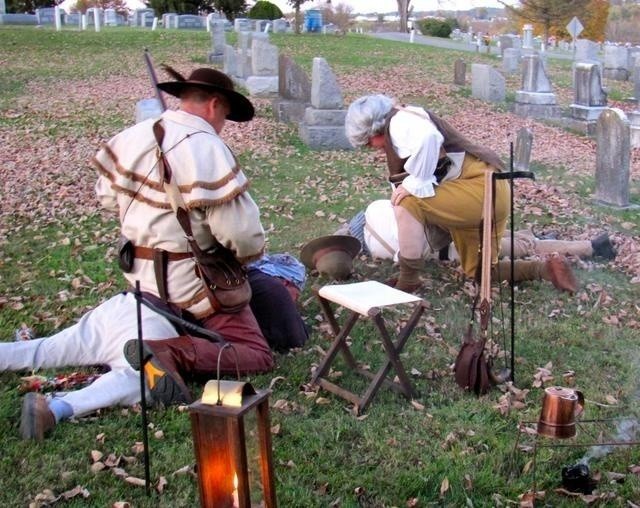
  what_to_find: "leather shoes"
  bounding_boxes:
[20,391,57,446]
[596,233,616,260]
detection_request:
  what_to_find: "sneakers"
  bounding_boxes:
[392,279,424,293]
[547,256,578,294]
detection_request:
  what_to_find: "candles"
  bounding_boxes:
[232,472,239,508]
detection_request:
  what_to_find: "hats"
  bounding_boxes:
[156,69,254,122]
[300,236,362,280]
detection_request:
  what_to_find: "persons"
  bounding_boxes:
[92,68,277,407]
[343,93,580,295]
[0,253,307,449]
[333,199,618,260]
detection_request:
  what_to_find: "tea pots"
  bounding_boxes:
[537,385,586,439]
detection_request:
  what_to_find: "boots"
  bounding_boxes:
[123,336,197,407]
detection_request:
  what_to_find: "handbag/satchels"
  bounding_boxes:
[196,249,254,315]
[455,339,489,395]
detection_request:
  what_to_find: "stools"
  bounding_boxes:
[310,280,430,416]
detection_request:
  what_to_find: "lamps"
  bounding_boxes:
[188,343,276,508]
